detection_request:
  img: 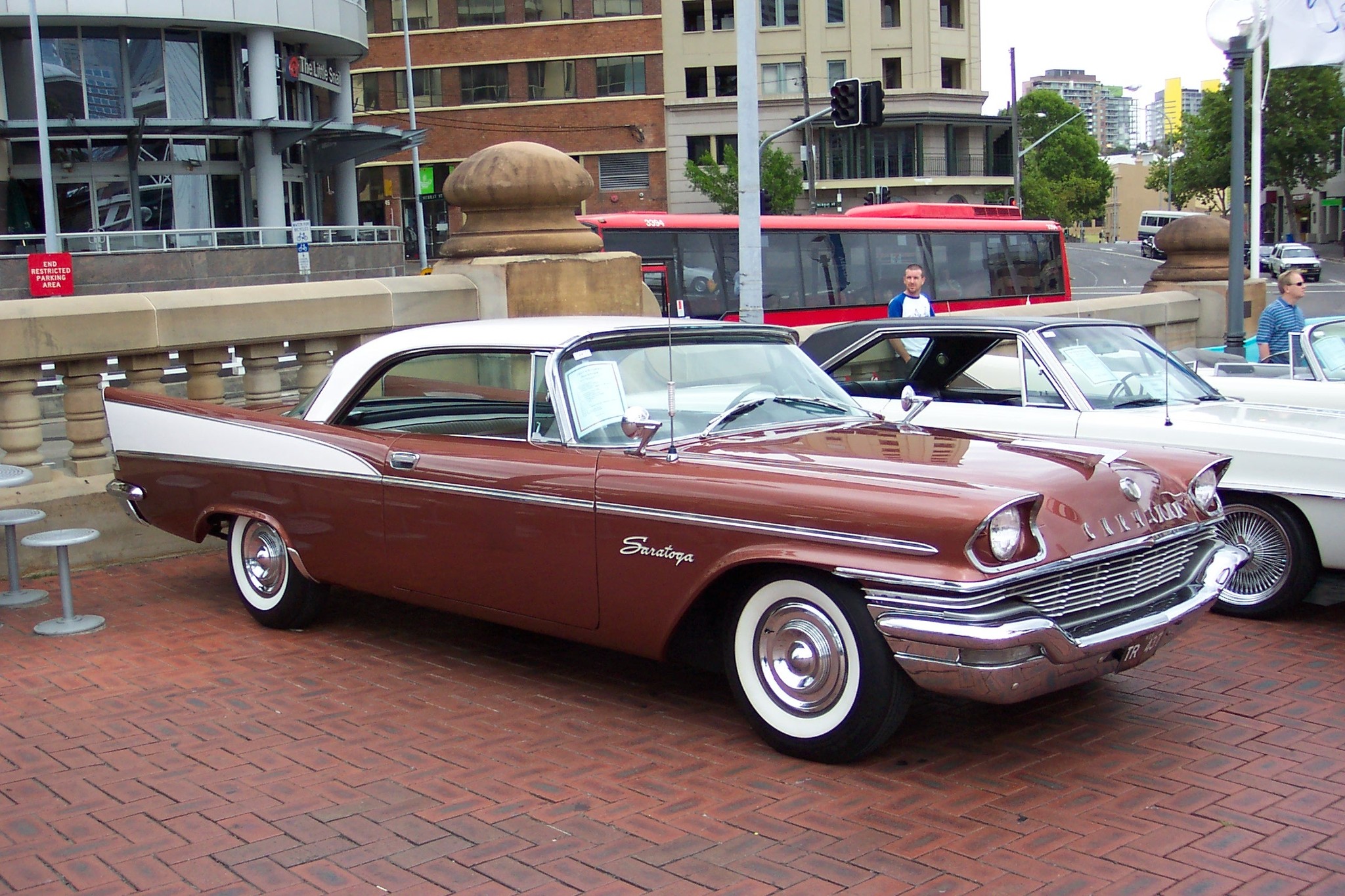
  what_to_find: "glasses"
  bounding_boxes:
[1288,282,1304,286]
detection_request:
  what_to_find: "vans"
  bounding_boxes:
[1138,210,1209,242]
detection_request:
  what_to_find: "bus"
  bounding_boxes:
[574,203,1073,329]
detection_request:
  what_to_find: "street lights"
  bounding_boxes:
[1013,84,1139,207]
[1130,107,1174,211]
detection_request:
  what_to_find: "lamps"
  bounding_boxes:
[187,158,203,168]
[60,160,74,172]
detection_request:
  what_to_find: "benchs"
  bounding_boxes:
[1175,347,1247,376]
[359,413,556,438]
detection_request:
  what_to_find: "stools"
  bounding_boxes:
[0,508,50,606]
[20,527,105,636]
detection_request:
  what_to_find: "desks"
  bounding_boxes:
[0,463,33,487]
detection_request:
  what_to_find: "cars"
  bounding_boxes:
[1171,315,1345,370]
[1243,240,1321,282]
[619,317,1345,623]
[644,265,714,294]
[1141,236,1167,260]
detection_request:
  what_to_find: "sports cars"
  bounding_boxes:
[957,319,1345,413]
[103,316,1250,765]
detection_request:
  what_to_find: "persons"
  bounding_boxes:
[887,264,936,379]
[1256,269,1306,370]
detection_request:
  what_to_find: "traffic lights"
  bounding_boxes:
[830,78,862,128]
[880,186,891,204]
[1008,196,1022,216]
[862,80,885,127]
[863,192,875,206]
